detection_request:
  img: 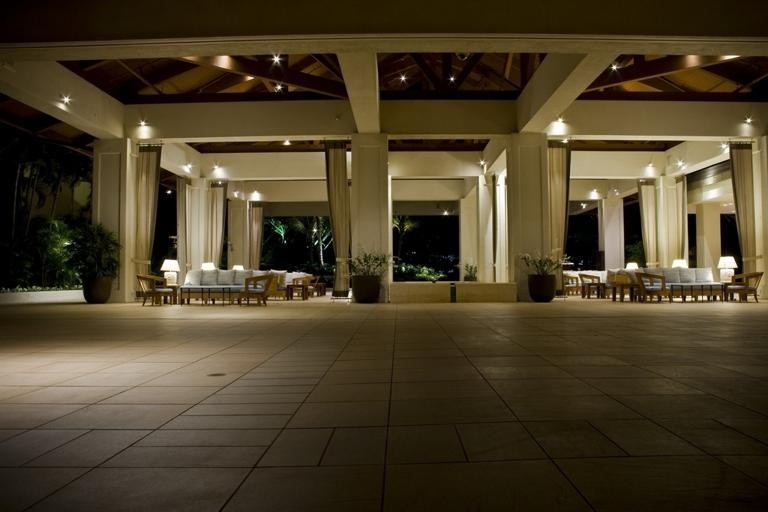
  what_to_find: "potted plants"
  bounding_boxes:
[62,218,125,303]
[462,263,478,281]
[344,251,391,303]
[519,251,572,301]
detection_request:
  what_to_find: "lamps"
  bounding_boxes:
[232,264,244,271]
[671,258,688,269]
[624,262,639,270]
[163,271,178,281]
[160,259,180,286]
[716,256,738,283]
[199,262,217,270]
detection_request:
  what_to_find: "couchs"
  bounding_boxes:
[178,270,314,307]
[564,268,723,302]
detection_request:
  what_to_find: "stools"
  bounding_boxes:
[310,283,326,297]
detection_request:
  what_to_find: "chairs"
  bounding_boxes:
[293,276,320,298]
[136,269,177,306]
[722,271,764,302]
